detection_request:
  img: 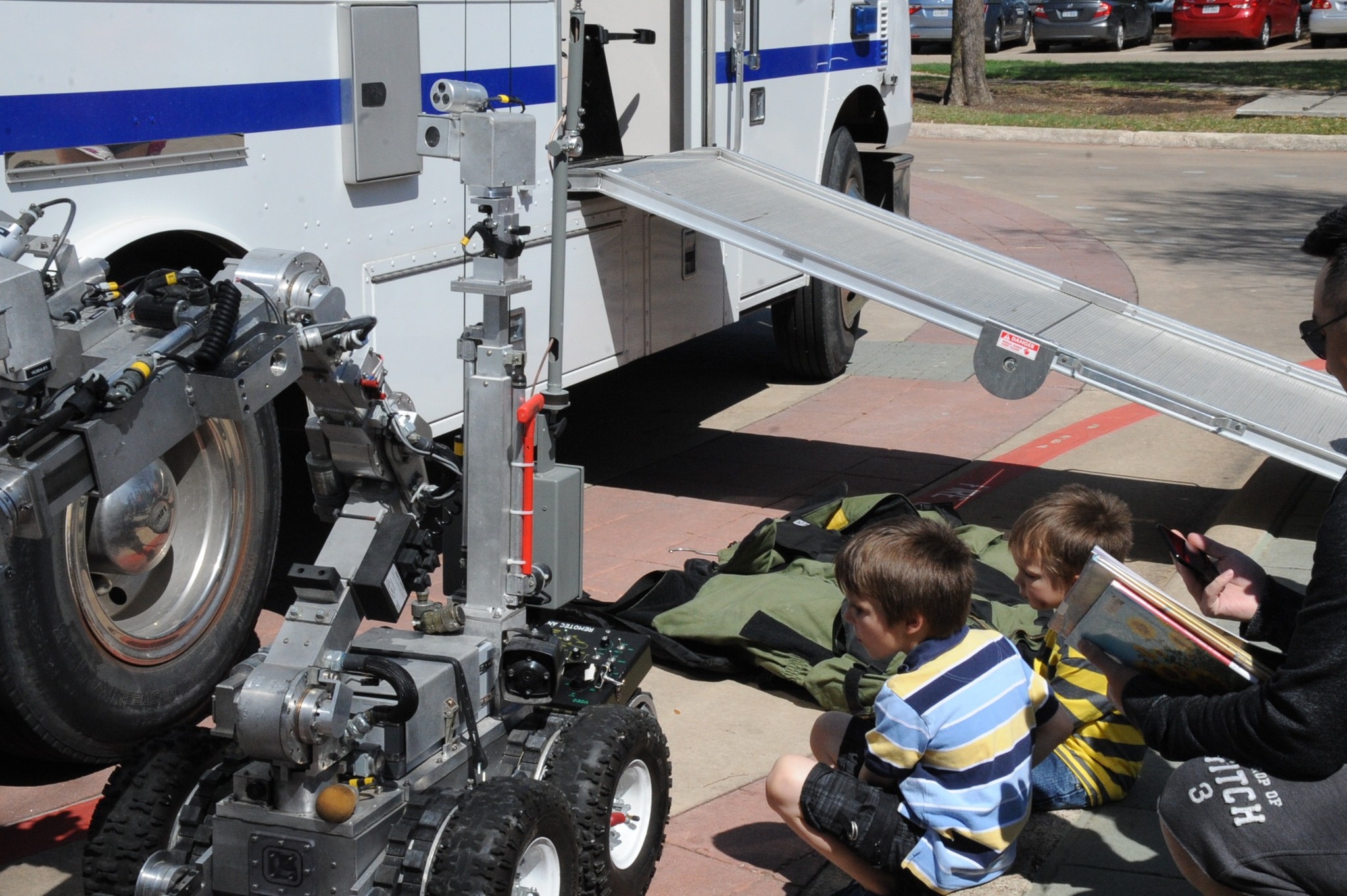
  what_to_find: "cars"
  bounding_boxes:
[1170,1,1347,52]
[981,0,1033,54]
[907,0,1034,53]
[1030,0,1158,52]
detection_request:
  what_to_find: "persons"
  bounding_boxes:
[1004,484,1148,812]
[1067,208,1347,896]
[767,517,1076,895]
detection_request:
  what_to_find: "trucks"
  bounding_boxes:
[0,0,929,770]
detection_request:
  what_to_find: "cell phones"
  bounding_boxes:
[1157,524,1220,582]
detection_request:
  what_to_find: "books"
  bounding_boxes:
[1045,546,1282,697]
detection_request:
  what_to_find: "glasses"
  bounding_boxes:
[1300,309,1347,359]
